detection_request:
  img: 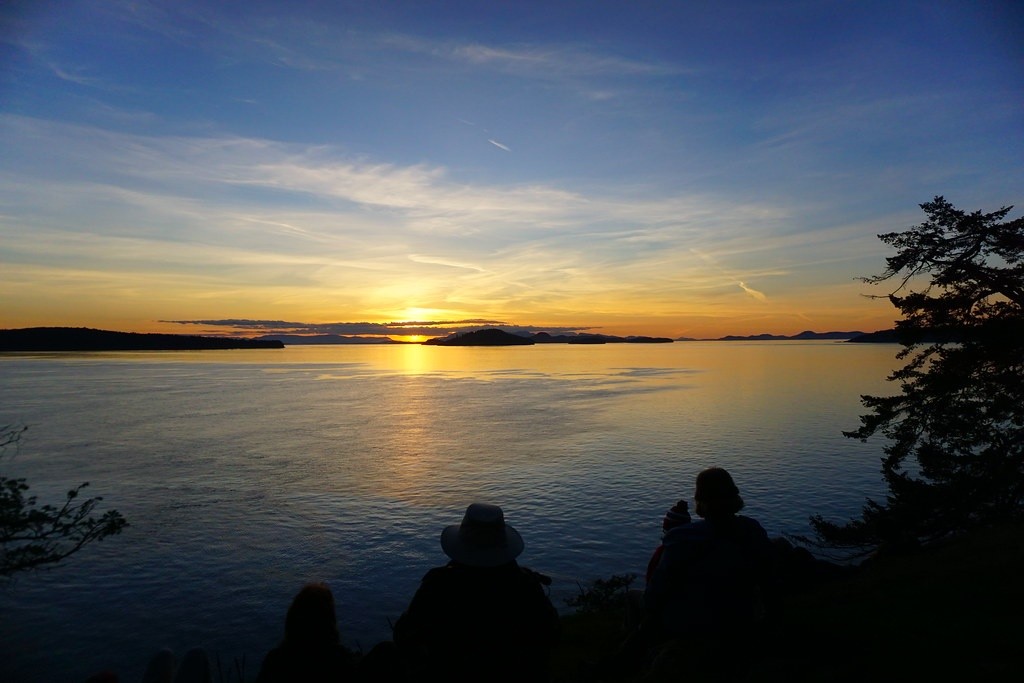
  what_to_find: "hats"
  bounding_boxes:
[440,503,524,568]
[663,500,691,533]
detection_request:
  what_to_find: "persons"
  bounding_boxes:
[644,469,817,636]
[258,585,358,683]
[393,505,556,683]
